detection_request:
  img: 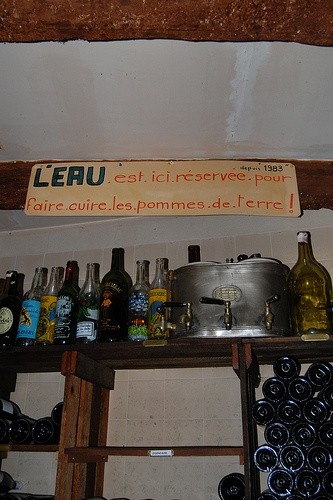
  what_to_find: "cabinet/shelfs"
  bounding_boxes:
[0,332,333,500]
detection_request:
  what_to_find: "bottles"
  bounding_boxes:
[251,356,332,500]
[216,473,245,500]
[1,399,64,500]
[0,232,333,347]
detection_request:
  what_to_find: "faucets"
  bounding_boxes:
[199,295,234,332]
[258,293,281,331]
[164,301,194,332]
